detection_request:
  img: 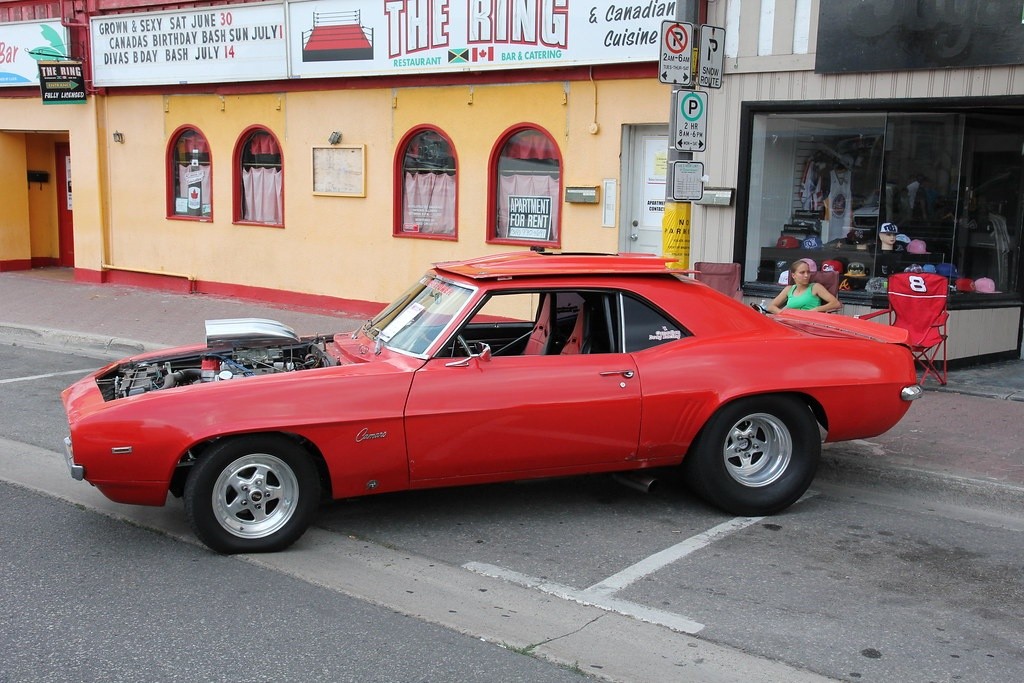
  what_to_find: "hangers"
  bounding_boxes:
[836,162,847,170]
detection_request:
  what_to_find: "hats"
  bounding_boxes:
[776,222,1004,294]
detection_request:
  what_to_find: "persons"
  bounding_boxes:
[769,262,842,313]
[879,220,898,250]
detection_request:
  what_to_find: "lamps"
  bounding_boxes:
[113,130,123,143]
[328,130,342,145]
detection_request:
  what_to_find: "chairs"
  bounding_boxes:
[560,302,598,357]
[524,294,558,356]
[859,272,950,386]
[694,262,744,301]
[788,271,840,314]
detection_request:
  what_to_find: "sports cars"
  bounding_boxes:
[60,247,923,557]
